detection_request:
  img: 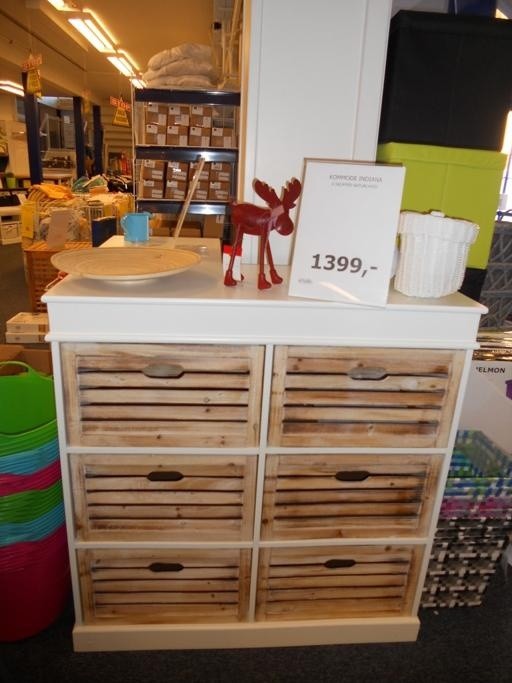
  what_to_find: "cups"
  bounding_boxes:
[120,213,149,243]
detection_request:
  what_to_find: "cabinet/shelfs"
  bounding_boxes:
[38,235,492,654]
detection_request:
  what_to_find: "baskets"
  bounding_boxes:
[392,207,480,298]
[418,428,512,609]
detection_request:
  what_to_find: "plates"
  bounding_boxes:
[49,246,202,286]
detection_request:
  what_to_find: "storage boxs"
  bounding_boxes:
[376,8,511,300]
[137,103,237,240]
